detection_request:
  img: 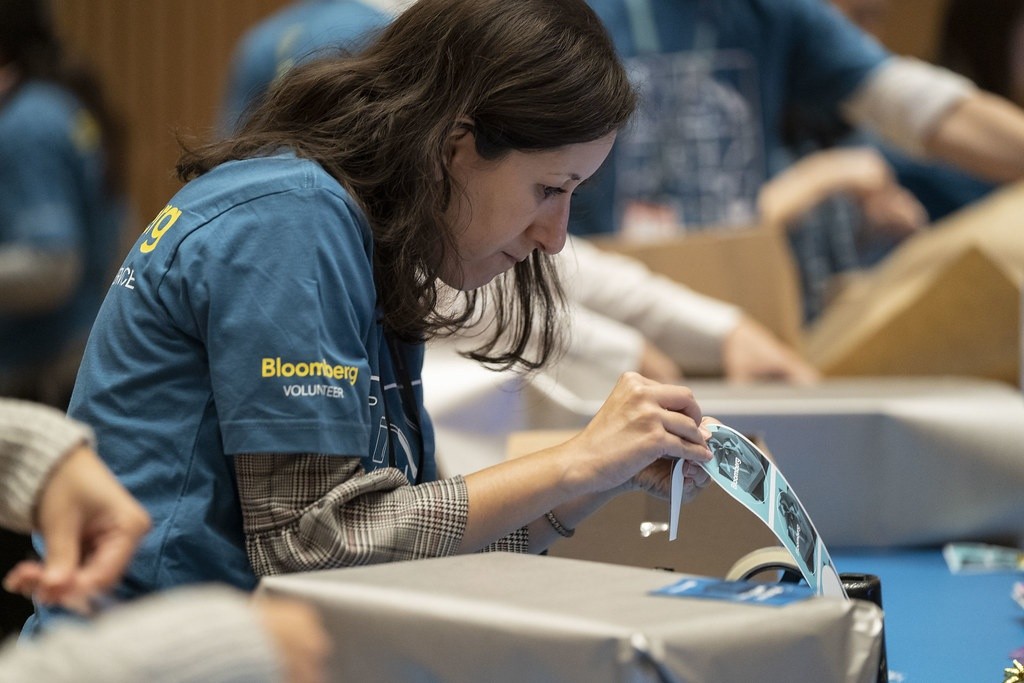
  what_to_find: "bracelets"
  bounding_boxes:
[547,510,575,538]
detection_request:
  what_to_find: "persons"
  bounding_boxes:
[0,0,123,412]
[12,0,712,655]
[0,399,336,682]
[565,0,1023,316]
[212,0,823,409]
